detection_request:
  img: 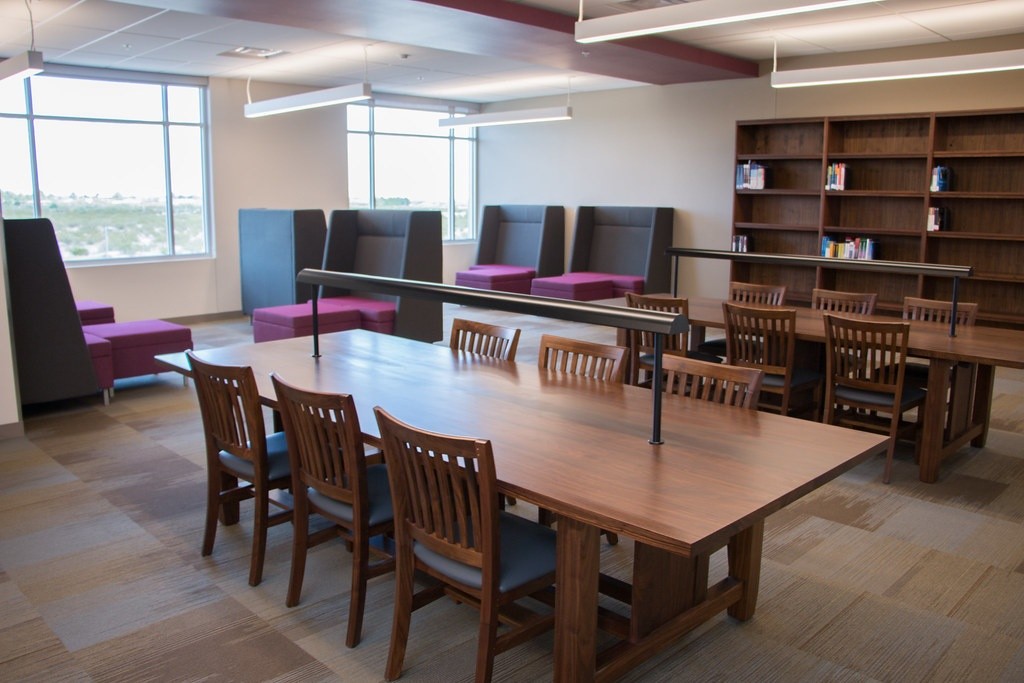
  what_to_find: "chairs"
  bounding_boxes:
[624,291,723,386]
[182,349,345,587]
[269,373,430,649]
[822,314,929,484]
[811,287,879,416]
[874,296,979,411]
[449,318,521,360]
[505,334,631,545]
[374,405,559,683]
[696,281,787,363]
[660,351,765,414]
[721,302,826,423]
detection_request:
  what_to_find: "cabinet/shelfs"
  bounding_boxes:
[728,107,1024,330]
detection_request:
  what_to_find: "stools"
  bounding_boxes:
[530,276,615,301]
[455,268,530,294]
[253,303,363,343]
[80,318,193,395]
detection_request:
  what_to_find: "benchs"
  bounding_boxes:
[563,270,645,296]
[308,294,396,333]
[469,263,536,286]
[74,301,115,406]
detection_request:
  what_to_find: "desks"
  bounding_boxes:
[153,328,890,683]
[589,293,1024,484]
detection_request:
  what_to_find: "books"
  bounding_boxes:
[732,235,747,253]
[735,159,765,190]
[821,235,880,261]
[930,165,953,193]
[824,163,846,191]
[926,206,945,231]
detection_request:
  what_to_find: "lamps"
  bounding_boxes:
[243,51,373,120]
[573,0,846,45]
[769,42,1024,88]
[0,0,44,85]
[438,78,573,128]
